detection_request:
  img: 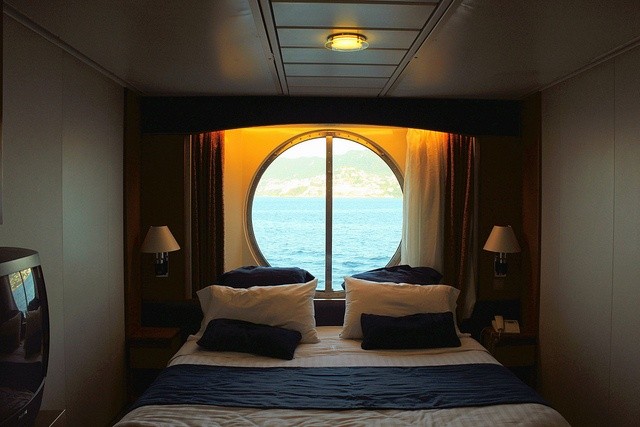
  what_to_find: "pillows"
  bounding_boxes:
[26,304,44,329]
[198,318,300,359]
[361,312,462,348]
[212,263,315,287]
[198,277,320,343]
[341,264,442,292]
[339,275,466,340]
[4,311,23,347]
[25,325,45,357]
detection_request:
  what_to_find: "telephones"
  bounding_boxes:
[491,314,519,334]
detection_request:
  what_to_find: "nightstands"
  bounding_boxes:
[483,325,535,383]
[128,327,181,402]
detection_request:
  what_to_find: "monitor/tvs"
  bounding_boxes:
[0,246,49,427]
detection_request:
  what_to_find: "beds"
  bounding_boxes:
[113,324,543,427]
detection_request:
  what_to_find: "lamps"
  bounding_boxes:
[483,225,522,277]
[140,224,181,278]
[324,31,368,51]
